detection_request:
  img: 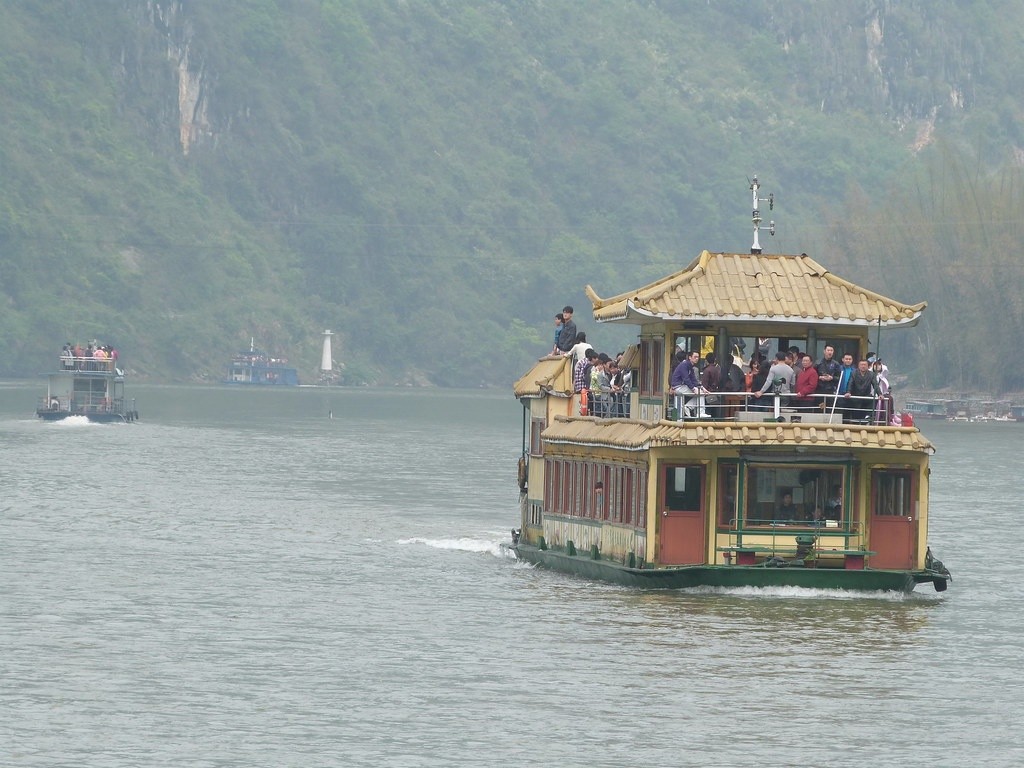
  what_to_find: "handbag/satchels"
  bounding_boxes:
[705,395,720,405]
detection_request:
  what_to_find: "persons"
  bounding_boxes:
[827,485,842,506]
[62,342,117,371]
[806,506,822,524]
[549,313,565,355]
[667,336,890,426]
[574,349,631,417]
[558,305,576,355]
[774,493,799,523]
[595,481,603,494]
[564,332,593,362]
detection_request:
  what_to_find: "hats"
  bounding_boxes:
[868,357,880,365]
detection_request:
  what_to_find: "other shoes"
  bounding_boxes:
[684,406,691,417]
[697,413,711,418]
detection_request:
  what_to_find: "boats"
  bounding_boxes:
[216,336,301,388]
[503,173,954,600]
[34,339,139,423]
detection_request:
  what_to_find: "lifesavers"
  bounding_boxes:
[126,409,139,422]
[580,388,588,416]
[517,457,526,485]
